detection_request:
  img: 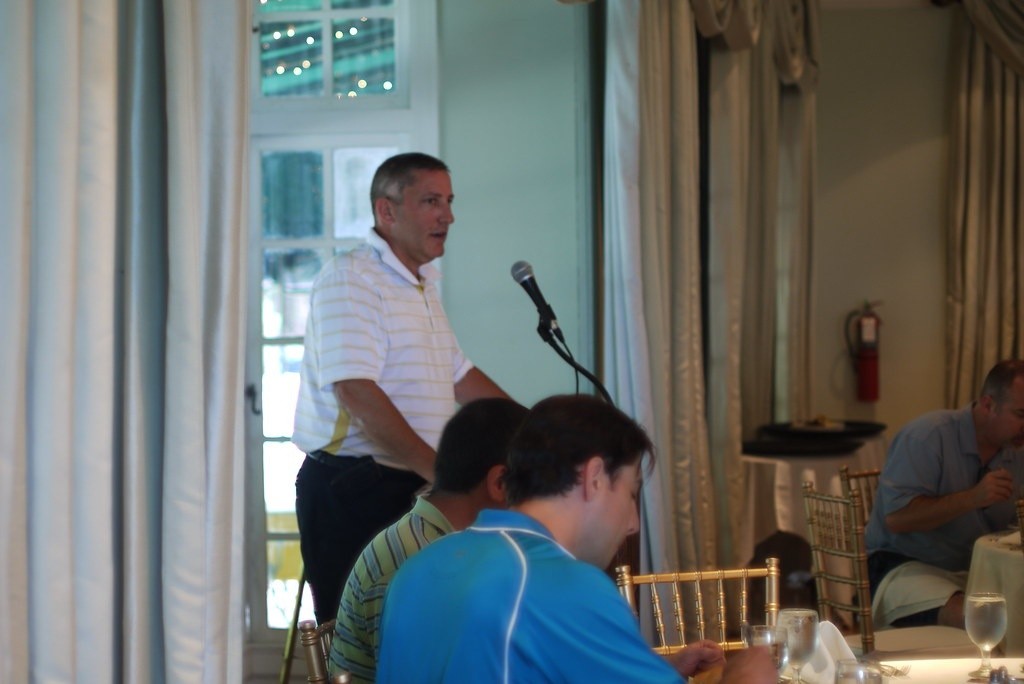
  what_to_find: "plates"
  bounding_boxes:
[742,421,887,456]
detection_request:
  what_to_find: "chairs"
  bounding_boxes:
[798,466,1003,660]
[294,618,338,684]
[616,558,779,659]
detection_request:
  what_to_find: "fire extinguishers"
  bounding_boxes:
[845,302,884,404]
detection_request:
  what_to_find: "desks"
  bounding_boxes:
[965,529,1024,660]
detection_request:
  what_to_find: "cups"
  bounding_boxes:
[1014,499,1024,548]
[835,658,882,684]
[746,625,789,676]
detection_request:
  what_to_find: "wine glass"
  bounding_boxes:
[965,592,1007,678]
[776,608,819,684]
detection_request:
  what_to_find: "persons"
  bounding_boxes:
[374,394,781,684]
[864,358,1024,628]
[327,397,531,684]
[291,153,513,625]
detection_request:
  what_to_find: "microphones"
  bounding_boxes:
[511,260,565,344]
